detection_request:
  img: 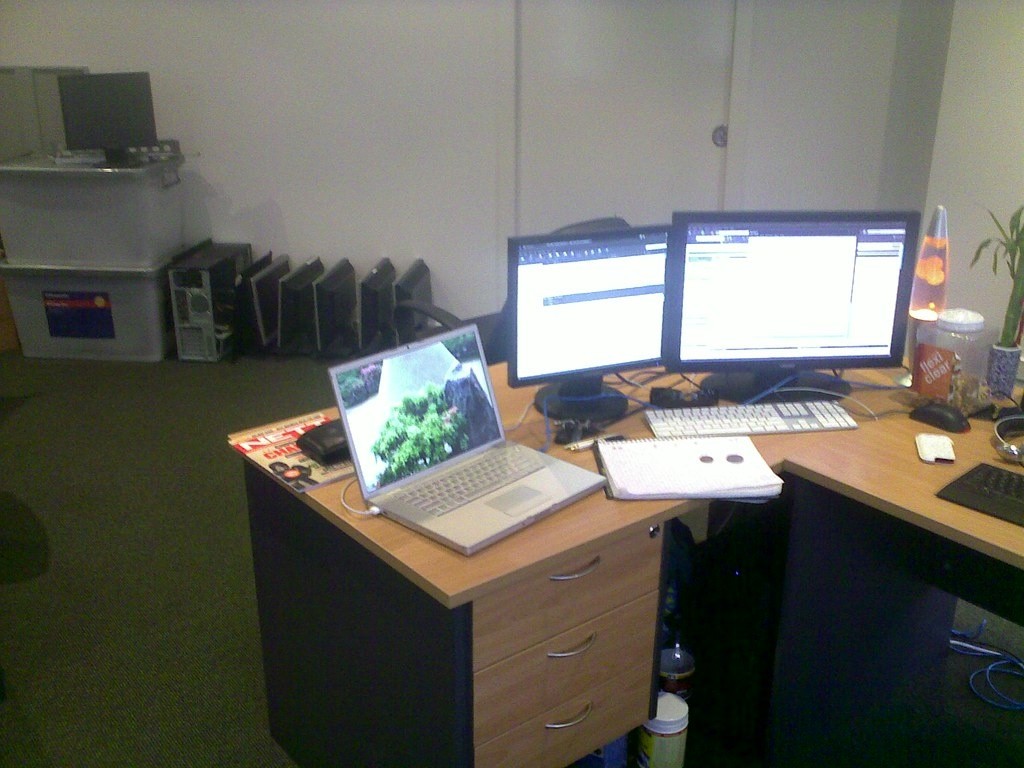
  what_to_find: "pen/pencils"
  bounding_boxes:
[563,432,625,452]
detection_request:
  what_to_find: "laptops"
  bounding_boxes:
[329,323,606,556]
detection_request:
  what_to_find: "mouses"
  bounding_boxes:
[910,402,971,434]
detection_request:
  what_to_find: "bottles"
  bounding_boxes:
[936,308,986,410]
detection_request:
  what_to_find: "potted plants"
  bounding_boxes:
[969,205,1024,402]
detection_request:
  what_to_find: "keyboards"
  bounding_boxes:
[936,463,1024,527]
[643,399,858,439]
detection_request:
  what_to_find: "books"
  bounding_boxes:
[596,436,784,501]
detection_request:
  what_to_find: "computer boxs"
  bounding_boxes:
[168,242,253,360]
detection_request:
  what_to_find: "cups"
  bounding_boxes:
[659,648,696,700]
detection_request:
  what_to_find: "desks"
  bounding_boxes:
[227,357,1024,768]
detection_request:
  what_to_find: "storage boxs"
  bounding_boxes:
[0,151,202,266]
[0,238,212,363]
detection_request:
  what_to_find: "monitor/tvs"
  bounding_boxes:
[229,250,432,354]
[667,210,921,405]
[507,225,673,422]
[58,71,158,169]
